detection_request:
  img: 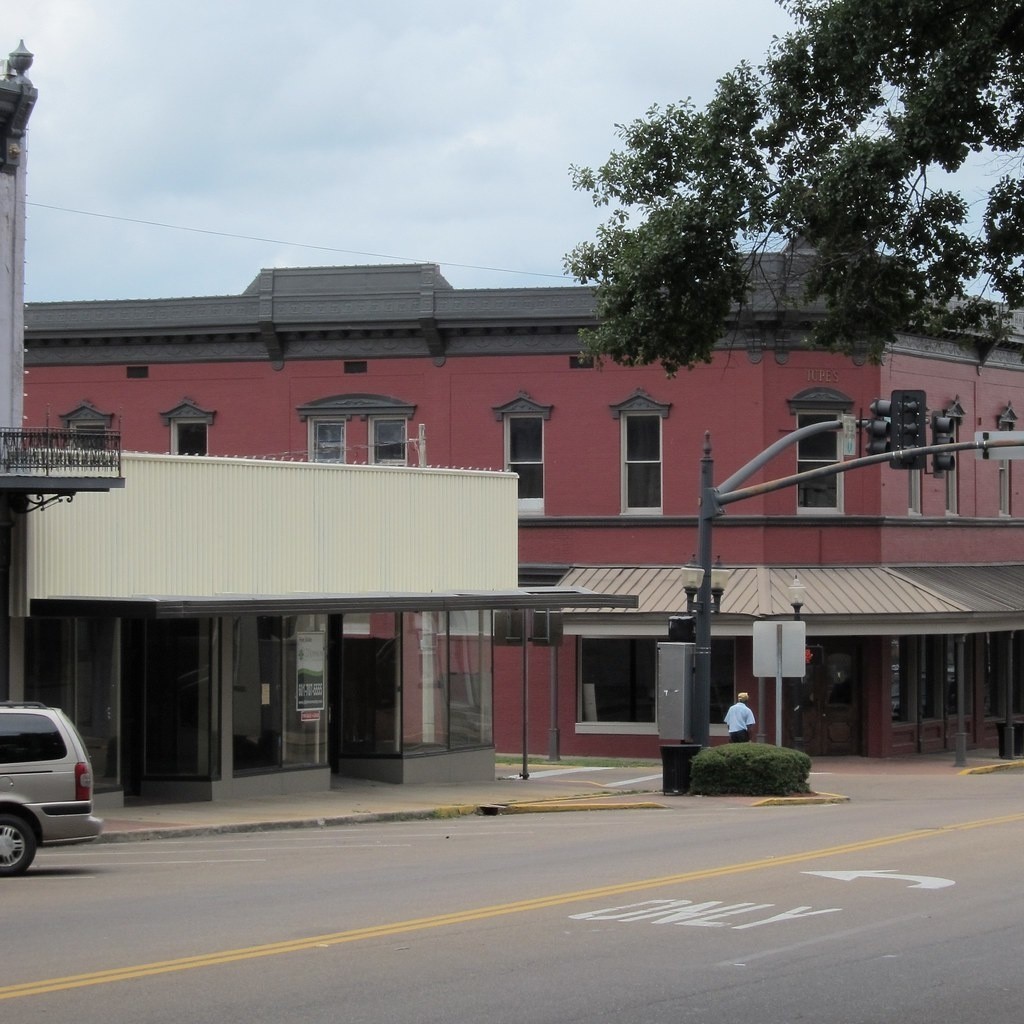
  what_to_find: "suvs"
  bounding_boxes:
[0,700,106,878]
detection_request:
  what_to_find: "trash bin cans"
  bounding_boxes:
[995,721,1024,758]
[660,744,700,796]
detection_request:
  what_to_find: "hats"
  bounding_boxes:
[738,692,749,702]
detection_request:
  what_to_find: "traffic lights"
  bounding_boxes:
[865,397,891,455]
[931,410,956,479]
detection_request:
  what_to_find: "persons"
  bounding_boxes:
[724,693,756,743]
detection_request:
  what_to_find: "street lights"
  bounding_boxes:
[784,574,808,752]
[678,429,734,752]
